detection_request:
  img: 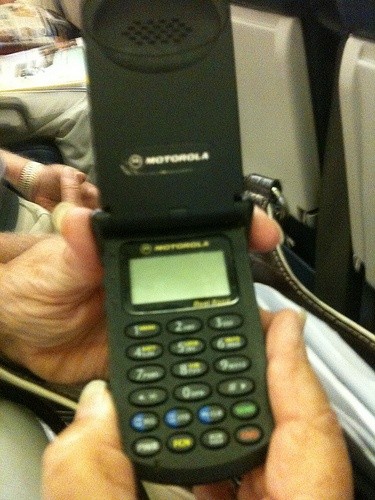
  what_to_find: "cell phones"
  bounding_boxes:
[80,0,276,486]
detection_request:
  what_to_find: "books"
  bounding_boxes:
[0,4,86,92]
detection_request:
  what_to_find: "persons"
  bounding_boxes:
[1,0,100,264]
[0,204,353,500]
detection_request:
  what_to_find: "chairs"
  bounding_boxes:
[228,3,375,289]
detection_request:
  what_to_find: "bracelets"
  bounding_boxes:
[18,160,44,200]
